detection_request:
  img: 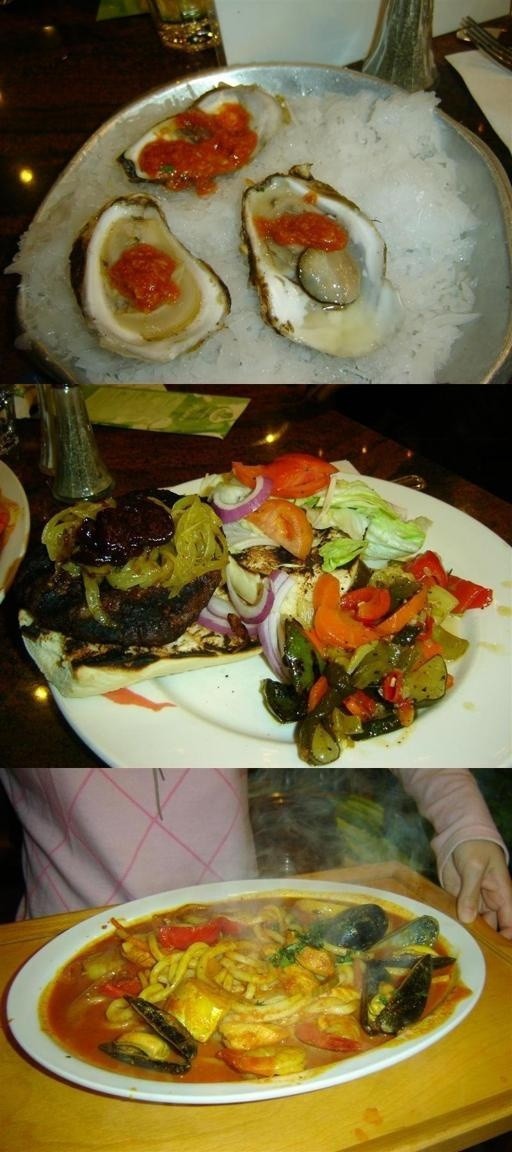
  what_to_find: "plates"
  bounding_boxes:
[3,873,487,1109]
[32,467,511,766]
[17,59,511,383]
[0,455,31,609]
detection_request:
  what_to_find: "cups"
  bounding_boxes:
[146,1,226,55]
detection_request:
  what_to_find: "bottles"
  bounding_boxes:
[363,1,445,93]
[39,386,110,501]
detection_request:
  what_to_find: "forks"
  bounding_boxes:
[459,14,511,73]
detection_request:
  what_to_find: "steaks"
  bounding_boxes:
[16,486,227,648]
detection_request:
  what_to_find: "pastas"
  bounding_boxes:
[106,922,442,1027]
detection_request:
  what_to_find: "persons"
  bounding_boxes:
[2,767,512,947]
